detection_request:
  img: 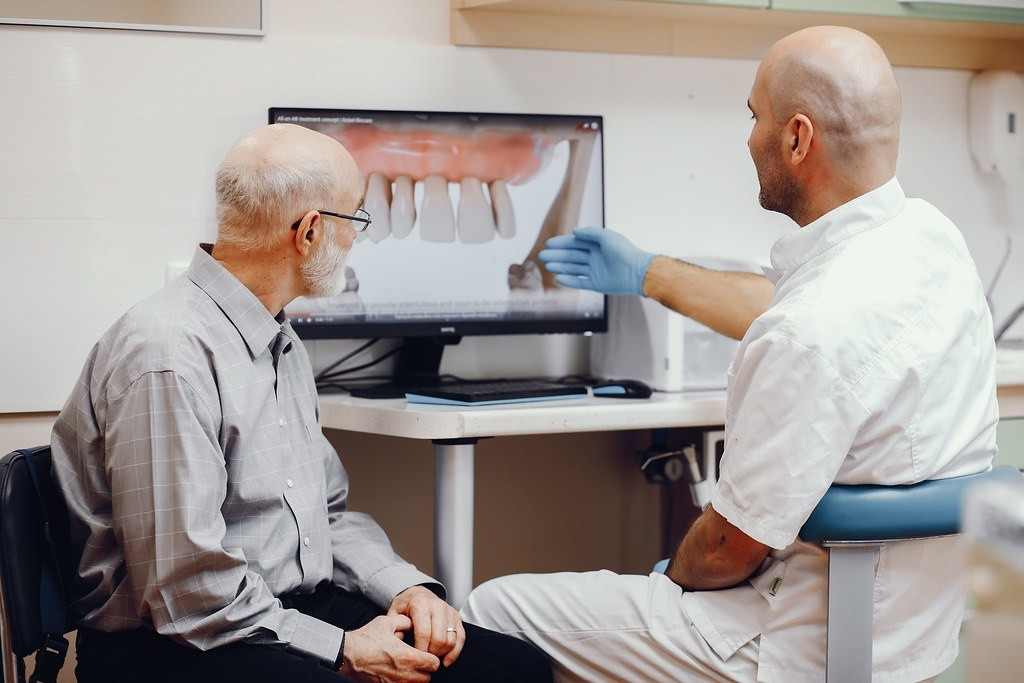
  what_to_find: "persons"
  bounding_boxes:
[48,124,555,683]
[455,26,999,683]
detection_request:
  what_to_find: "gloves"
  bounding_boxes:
[653,558,671,575]
[538,226,659,301]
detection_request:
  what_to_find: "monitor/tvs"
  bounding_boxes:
[266,107,604,396]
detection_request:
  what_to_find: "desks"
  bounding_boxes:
[316,390,727,612]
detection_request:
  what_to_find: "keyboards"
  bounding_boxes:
[405,377,587,402]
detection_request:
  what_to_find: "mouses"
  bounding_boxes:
[591,379,652,399]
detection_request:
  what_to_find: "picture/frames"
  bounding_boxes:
[0,0,268,38]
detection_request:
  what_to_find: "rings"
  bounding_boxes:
[446,628,457,633]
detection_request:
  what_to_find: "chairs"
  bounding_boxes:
[800,463,1024,683]
[0,445,91,683]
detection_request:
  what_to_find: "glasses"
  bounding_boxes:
[291,207,373,234]
